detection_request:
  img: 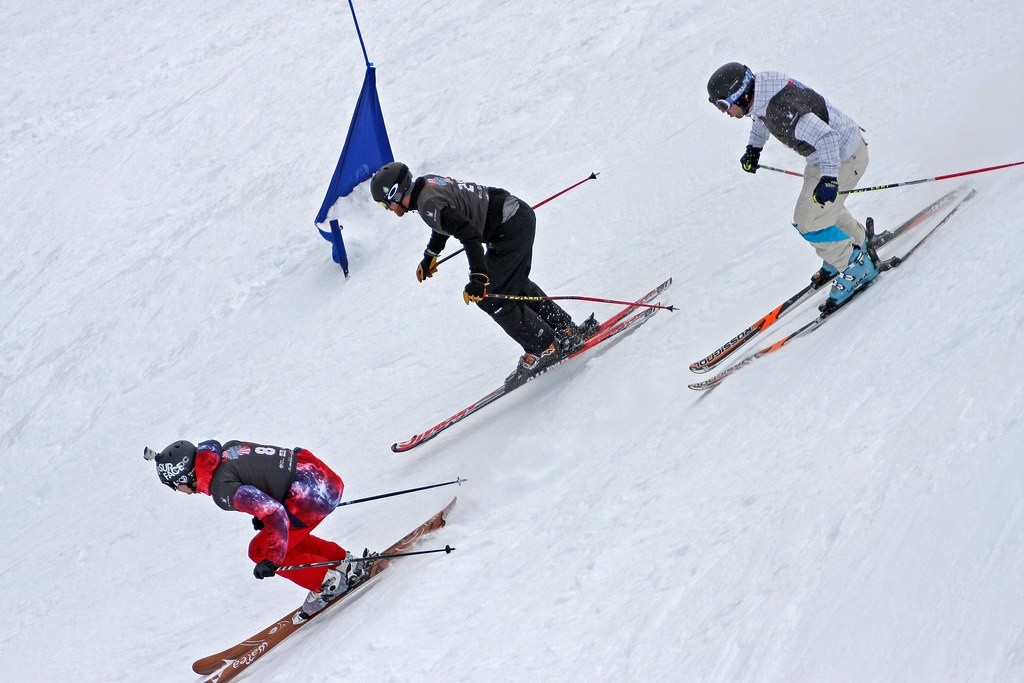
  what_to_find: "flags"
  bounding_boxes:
[316,63,394,222]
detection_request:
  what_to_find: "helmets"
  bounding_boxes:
[707,62,755,110]
[154,440,196,485]
[371,162,413,204]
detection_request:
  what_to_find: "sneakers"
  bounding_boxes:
[829,253,879,305]
[302,570,350,616]
[554,321,582,351]
[517,336,570,374]
[812,245,863,284]
[335,550,365,584]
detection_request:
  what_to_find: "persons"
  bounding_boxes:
[370,162,583,377]
[707,61,878,315]
[156,439,364,616]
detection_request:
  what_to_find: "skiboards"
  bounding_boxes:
[191,495,458,683]
[686,178,977,391]
[390,276,674,453]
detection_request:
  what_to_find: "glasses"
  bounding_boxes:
[168,482,179,491]
[714,100,731,113]
[377,201,389,210]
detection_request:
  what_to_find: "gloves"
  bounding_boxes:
[416,249,441,283]
[813,176,839,205]
[740,145,763,173]
[464,274,487,306]
[252,516,264,530]
[253,559,275,580]
